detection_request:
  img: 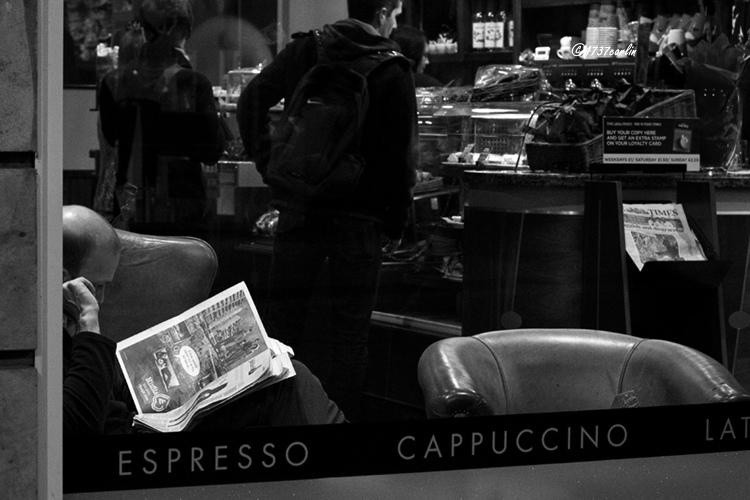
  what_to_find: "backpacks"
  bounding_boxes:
[267,29,403,205]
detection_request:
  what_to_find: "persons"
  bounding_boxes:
[62,203,350,440]
[95,1,233,237]
[235,0,420,423]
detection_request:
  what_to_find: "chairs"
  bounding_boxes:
[99,226,218,341]
[417,328,750,422]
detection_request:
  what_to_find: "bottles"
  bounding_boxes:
[472,0,514,52]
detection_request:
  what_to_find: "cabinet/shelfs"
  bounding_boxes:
[402,0,594,87]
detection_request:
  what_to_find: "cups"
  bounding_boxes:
[583,27,618,60]
